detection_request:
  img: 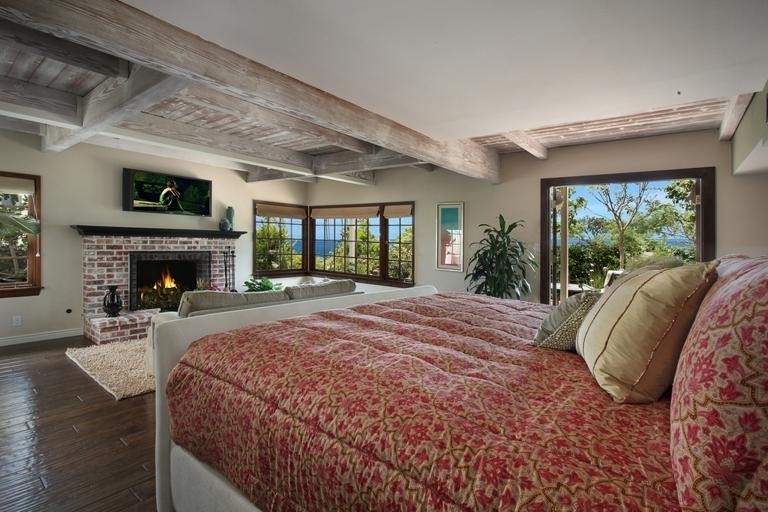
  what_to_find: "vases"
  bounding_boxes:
[219,218,230,231]
[103,286,122,317]
[226,207,234,230]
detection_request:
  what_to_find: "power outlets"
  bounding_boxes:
[12,315,22,326]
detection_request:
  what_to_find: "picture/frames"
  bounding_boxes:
[123,168,212,217]
[435,202,463,272]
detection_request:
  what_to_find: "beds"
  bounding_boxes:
[154,254,768,512]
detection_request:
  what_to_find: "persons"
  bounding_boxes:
[159,177,183,210]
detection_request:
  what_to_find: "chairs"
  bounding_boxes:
[579,270,622,293]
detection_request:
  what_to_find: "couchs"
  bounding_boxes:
[146,279,365,376]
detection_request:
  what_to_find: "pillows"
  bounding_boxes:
[285,279,356,300]
[668,253,767,512]
[530,290,602,351]
[178,290,289,318]
[576,261,719,407]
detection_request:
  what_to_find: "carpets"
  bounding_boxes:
[65,337,156,400]
